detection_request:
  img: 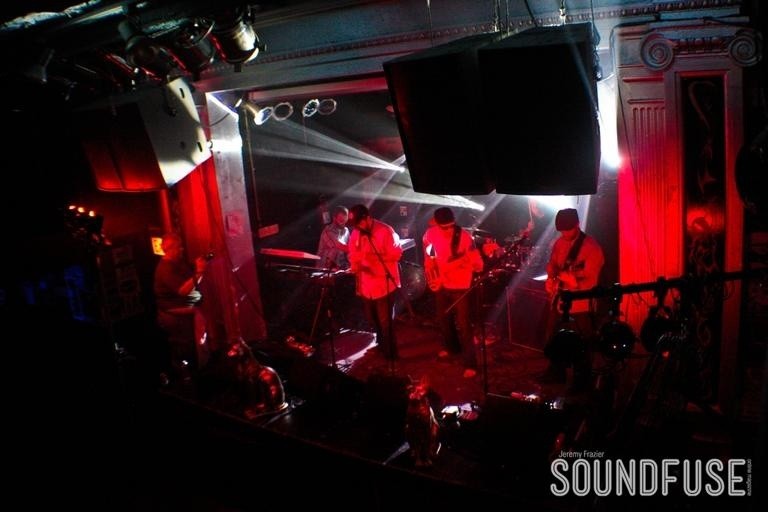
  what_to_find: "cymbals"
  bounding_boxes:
[463,226,491,233]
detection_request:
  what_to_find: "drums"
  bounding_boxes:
[398,261,426,302]
[519,246,549,268]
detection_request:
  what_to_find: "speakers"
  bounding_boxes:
[470,393,562,469]
[479,21,601,196]
[71,72,214,196]
[382,29,518,195]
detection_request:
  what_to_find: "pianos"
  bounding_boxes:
[259,248,356,286]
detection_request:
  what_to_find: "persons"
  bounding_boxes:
[154,232,211,366]
[419,206,486,386]
[314,204,350,272]
[346,204,403,368]
[530,209,606,394]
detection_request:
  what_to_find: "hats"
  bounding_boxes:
[345,205,367,227]
[555,207,579,230]
[434,207,455,225]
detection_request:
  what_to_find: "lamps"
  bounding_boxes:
[22,8,263,112]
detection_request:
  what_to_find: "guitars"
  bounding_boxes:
[544,260,585,294]
